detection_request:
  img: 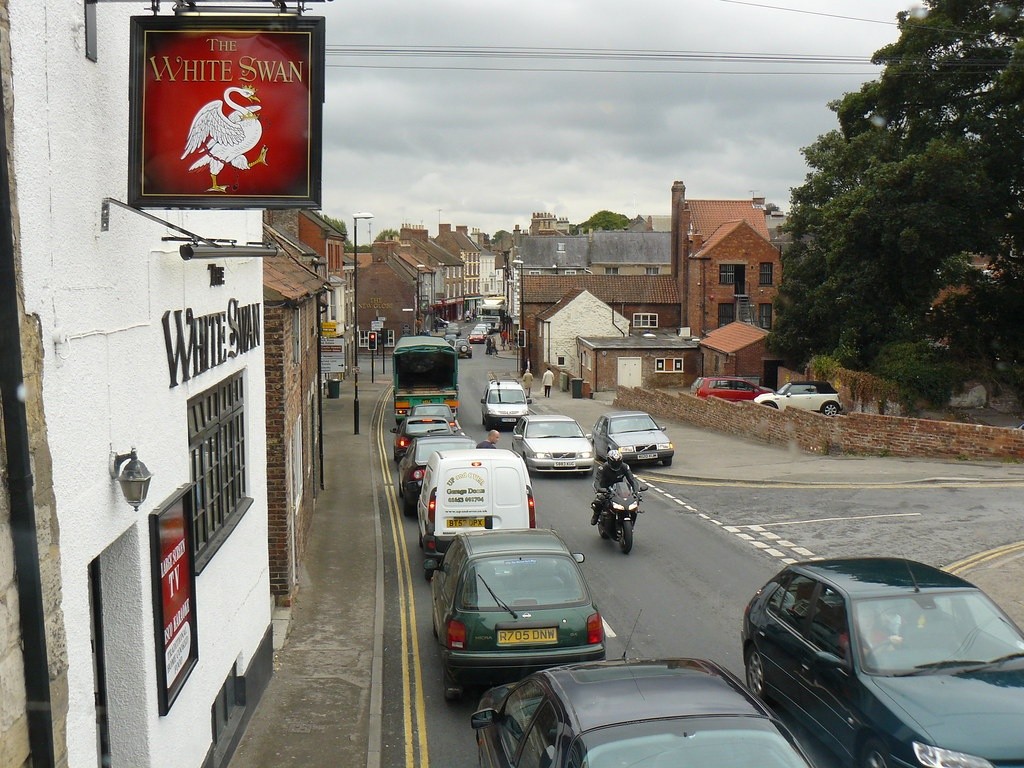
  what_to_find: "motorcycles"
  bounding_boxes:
[591,480,649,554]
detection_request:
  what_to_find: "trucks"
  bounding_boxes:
[392,336,459,425]
[478,305,505,332]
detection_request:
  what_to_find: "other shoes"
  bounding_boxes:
[591,512,600,526]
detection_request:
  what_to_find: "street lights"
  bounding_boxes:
[415,264,426,336]
[512,259,525,373]
[352,211,375,435]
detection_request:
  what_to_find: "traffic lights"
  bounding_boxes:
[518,330,526,348]
[368,332,377,350]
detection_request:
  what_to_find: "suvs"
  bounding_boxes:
[453,339,473,359]
[690,375,776,402]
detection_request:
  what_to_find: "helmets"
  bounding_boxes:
[607,450,623,472]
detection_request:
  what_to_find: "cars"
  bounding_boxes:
[390,403,480,519]
[434,317,494,346]
[422,527,607,702]
[470,654,821,768]
[589,411,674,470]
[741,552,1024,768]
[754,381,844,416]
[512,411,593,475]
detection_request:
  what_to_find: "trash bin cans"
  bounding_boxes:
[325,378,342,398]
[571,378,584,398]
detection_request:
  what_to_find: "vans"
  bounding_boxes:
[481,378,532,431]
[413,448,537,581]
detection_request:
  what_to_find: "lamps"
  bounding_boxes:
[101,198,284,259]
[110,443,154,513]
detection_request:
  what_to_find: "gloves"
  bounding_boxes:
[636,492,642,497]
[598,495,605,500]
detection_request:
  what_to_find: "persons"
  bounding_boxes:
[590,450,643,526]
[500,330,524,347]
[485,335,499,355]
[542,367,555,398]
[522,368,534,398]
[475,429,500,449]
